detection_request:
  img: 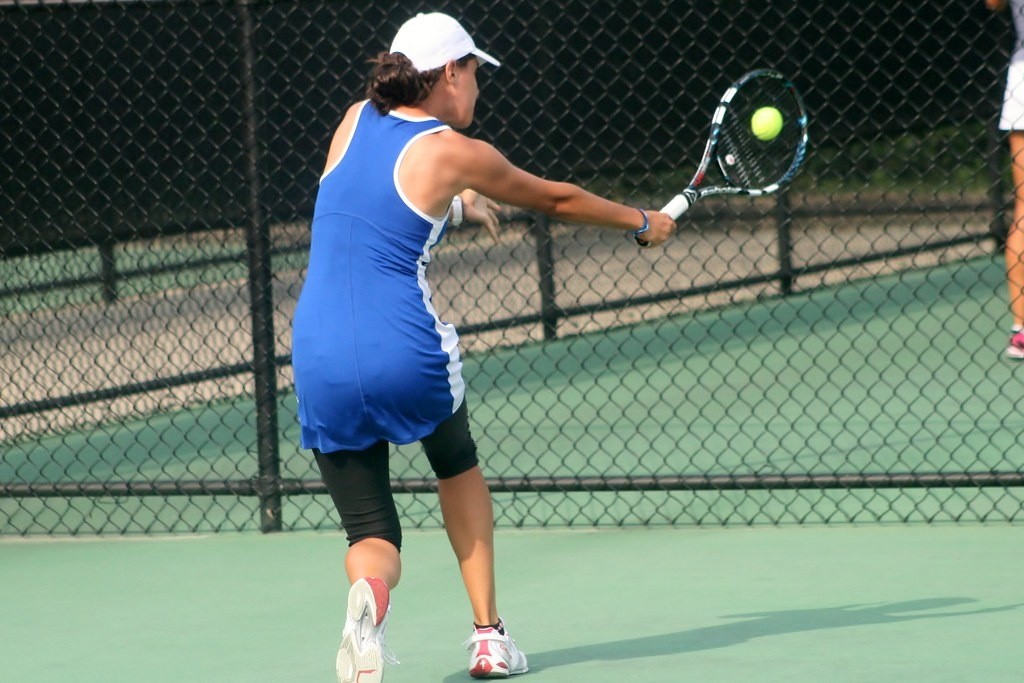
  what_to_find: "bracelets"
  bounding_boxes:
[451,195,463,226]
[630,209,649,235]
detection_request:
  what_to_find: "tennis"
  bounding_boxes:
[750,105,785,142]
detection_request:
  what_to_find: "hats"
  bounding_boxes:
[389,11,501,74]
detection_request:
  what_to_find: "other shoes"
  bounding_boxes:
[1005,328,1024,358]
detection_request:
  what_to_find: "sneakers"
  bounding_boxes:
[463,628,529,677]
[335,578,401,683]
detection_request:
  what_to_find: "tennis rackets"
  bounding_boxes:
[634,67,812,249]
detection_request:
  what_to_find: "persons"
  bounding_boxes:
[292,12,677,683]
[987,0,1024,358]
